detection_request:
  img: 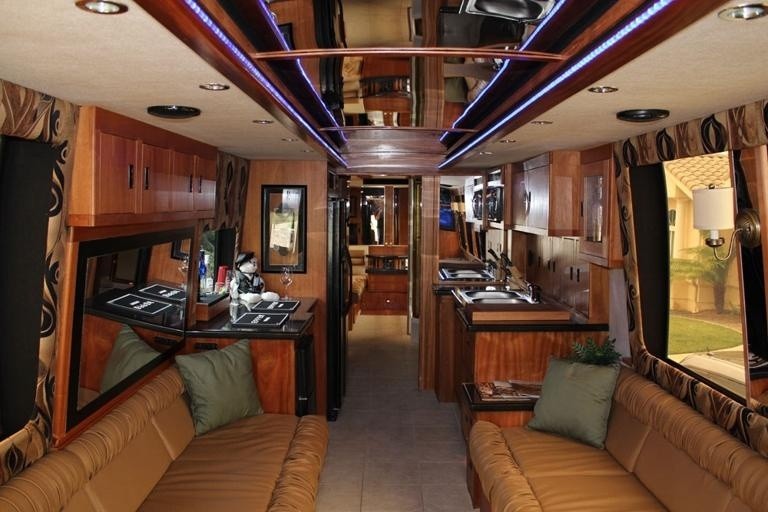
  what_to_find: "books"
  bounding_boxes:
[474,377,546,402]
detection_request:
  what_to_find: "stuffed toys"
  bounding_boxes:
[229,253,281,303]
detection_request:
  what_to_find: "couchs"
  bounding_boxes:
[3,352,328,511]
[467,350,767,509]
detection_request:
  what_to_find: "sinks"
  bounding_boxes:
[465,291,519,298]
[472,298,530,305]
[450,273,491,279]
[447,268,486,274]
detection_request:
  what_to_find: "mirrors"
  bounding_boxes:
[66,228,198,431]
[437,174,500,277]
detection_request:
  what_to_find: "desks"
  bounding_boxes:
[463,381,545,440]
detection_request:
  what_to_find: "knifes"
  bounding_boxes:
[484,249,497,270]
[499,253,513,277]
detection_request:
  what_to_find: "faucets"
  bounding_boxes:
[521,278,533,298]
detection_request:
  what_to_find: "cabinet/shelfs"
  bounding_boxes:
[66,134,172,215]
[171,151,219,212]
[559,235,591,317]
[456,314,481,412]
[508,151,583,234]
[578,161,625,270]
[530,237,560,299]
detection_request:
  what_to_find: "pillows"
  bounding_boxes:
[101,323,161,393]
[173,338,263,437]
[525,355,621,451]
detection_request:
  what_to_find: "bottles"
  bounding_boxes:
[228,298,239,323]
[199,250,206,296]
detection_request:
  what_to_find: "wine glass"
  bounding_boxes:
[279,266,294,300]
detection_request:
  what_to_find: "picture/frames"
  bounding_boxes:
[261,184,308,273]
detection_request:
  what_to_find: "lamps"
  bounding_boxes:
[694,184,761,261]
